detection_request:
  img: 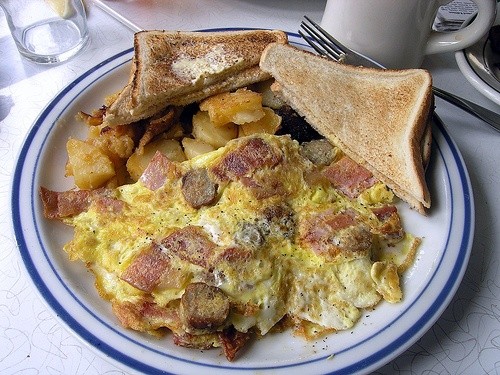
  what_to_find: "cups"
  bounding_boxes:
[320,0,494,70]
[0,0,88,65]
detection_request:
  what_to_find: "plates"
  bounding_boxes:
[454,2,500,106]
[12,28,475,375]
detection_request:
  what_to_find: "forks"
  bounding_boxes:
[299,15,500,139]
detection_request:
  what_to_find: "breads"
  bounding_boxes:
[258,42,431,216]
[102,29,290,127]
